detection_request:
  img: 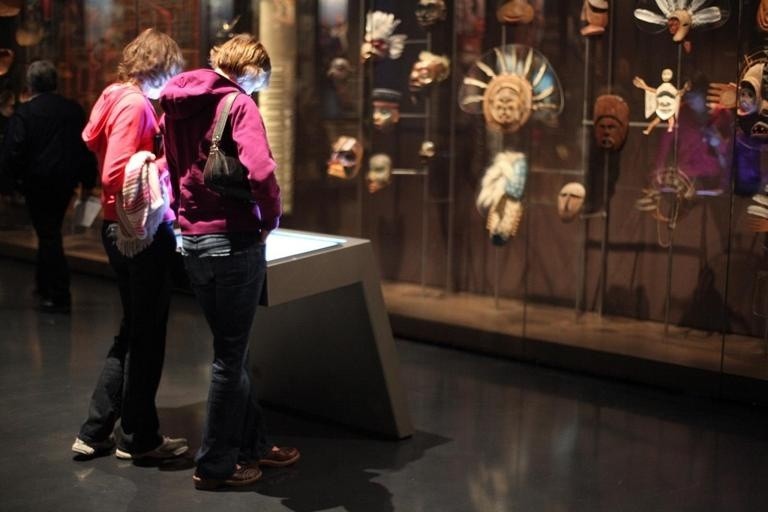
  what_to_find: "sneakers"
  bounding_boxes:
[72,433,119,455]
[115,436,188,458]
[238,445,301,467]
[192,461,263,490]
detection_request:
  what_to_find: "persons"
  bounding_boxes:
[158,31,301,487]
[10,57,100,322]
[68,24,192,465]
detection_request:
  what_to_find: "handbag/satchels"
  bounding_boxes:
[203,144,257,205]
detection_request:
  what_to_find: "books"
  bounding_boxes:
[70,197,105,228]
